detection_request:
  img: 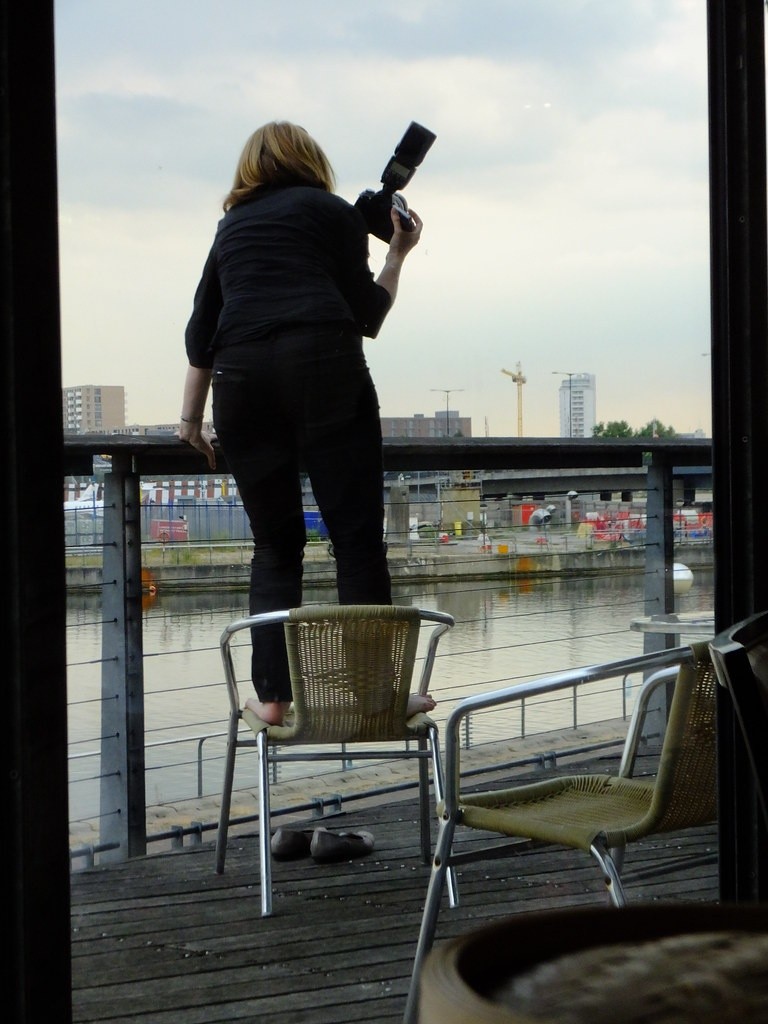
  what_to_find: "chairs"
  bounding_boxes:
[708,609,768,824]
[214,605,459,919]
[402,641,716,1023]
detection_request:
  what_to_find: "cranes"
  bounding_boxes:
[501,359,529,436]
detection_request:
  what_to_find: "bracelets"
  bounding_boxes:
[181,415,204,422]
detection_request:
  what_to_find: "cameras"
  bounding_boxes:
[354,121,437,243]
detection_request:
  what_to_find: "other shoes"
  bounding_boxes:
[270,827,316,859]
[308,825,376,865]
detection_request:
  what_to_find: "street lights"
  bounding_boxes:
[677,499,684,541]
[479,502,488,553]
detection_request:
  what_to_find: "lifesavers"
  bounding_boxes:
[158,532,170,543]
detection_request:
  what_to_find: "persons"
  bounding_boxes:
[174,122,437,724]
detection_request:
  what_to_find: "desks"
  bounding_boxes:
[629,610,714,635]
